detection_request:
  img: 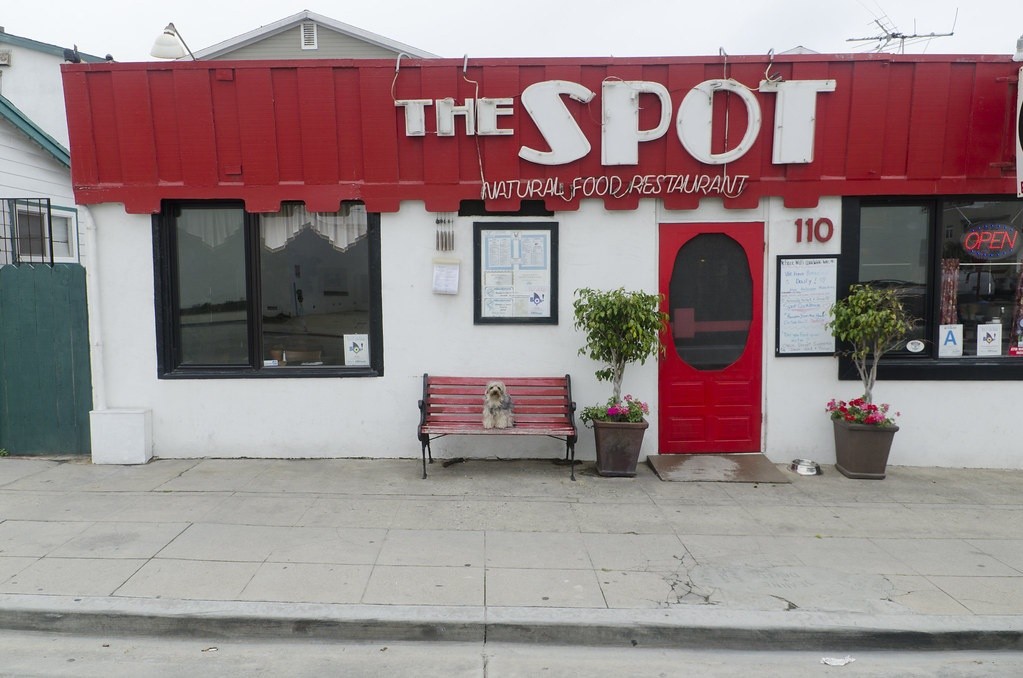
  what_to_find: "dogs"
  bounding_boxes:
[482,380,514,429]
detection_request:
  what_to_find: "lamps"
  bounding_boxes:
[150,22,196,62]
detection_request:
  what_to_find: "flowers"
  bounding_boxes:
[827,285,911,425]
[571,285,668,429]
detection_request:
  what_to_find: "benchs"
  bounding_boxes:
[417,373,578,479]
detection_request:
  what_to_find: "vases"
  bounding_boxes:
[592,417,650,476]
[831,417,899,479]
